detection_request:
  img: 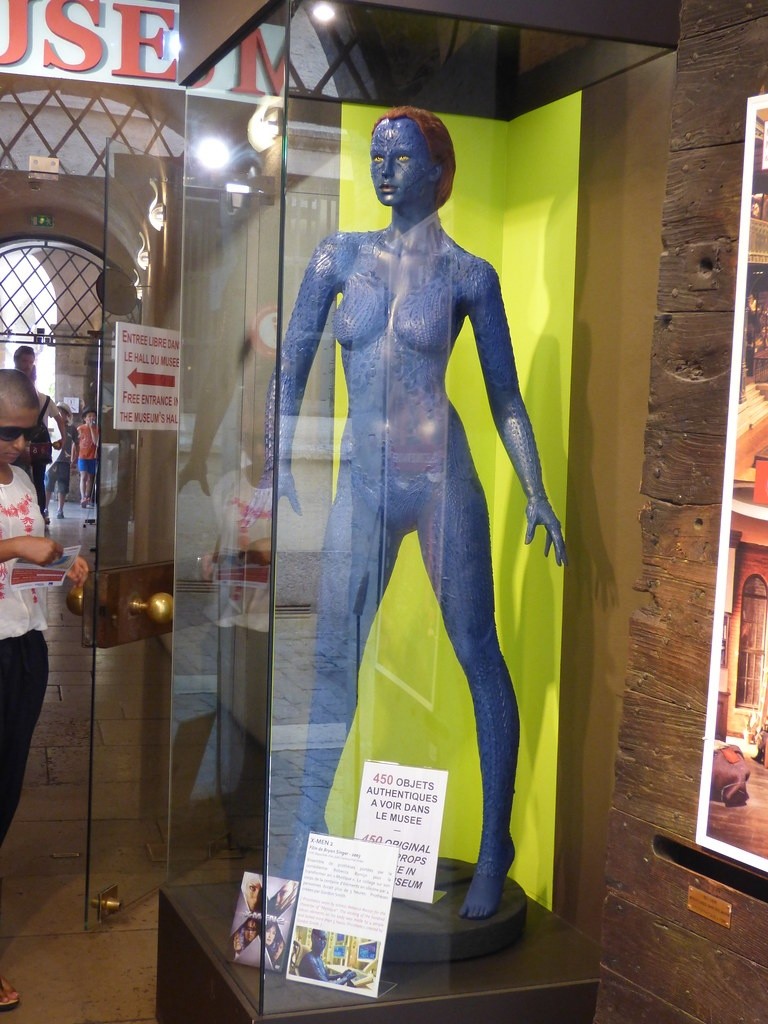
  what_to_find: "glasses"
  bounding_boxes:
[0,426,42,441]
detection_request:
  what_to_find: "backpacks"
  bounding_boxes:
[20,395,52,465]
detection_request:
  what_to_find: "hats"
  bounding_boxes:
[81,406,96,417]
[56,402,72,417]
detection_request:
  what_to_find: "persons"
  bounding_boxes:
[14,345,97,525]
[0,369,90,1012]
[298,928,357,986]
[231,872,298,970]
[240,106,569,920]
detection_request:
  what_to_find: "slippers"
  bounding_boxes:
[0,998,20,1012]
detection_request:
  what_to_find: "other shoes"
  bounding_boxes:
[81,501,86,508]
[57,508,64,519]
[44,509,49,517]
